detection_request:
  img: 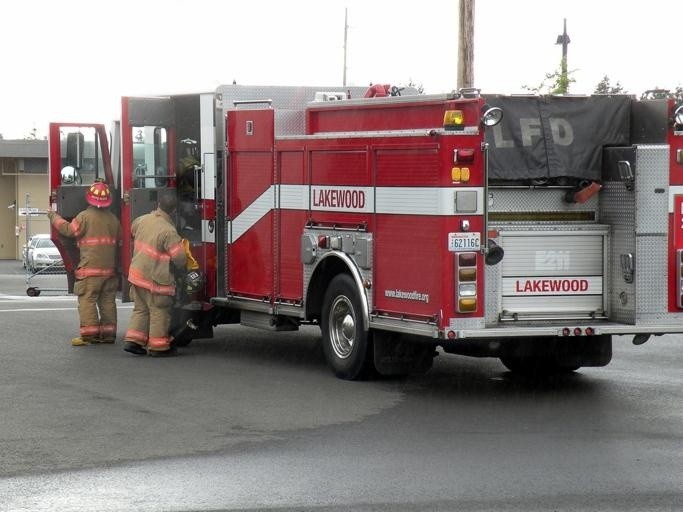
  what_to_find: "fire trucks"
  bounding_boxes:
[48,79,682,380]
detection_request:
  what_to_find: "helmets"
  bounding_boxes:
[85,182,114,208]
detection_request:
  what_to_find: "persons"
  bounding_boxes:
[122,190,188,359]
[175,141,201,195]
[45,180,123,347]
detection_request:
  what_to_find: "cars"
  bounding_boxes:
[21,234,66,275]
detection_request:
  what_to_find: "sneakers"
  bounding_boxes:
[71,331,115,346]
[124,340,177,357]
